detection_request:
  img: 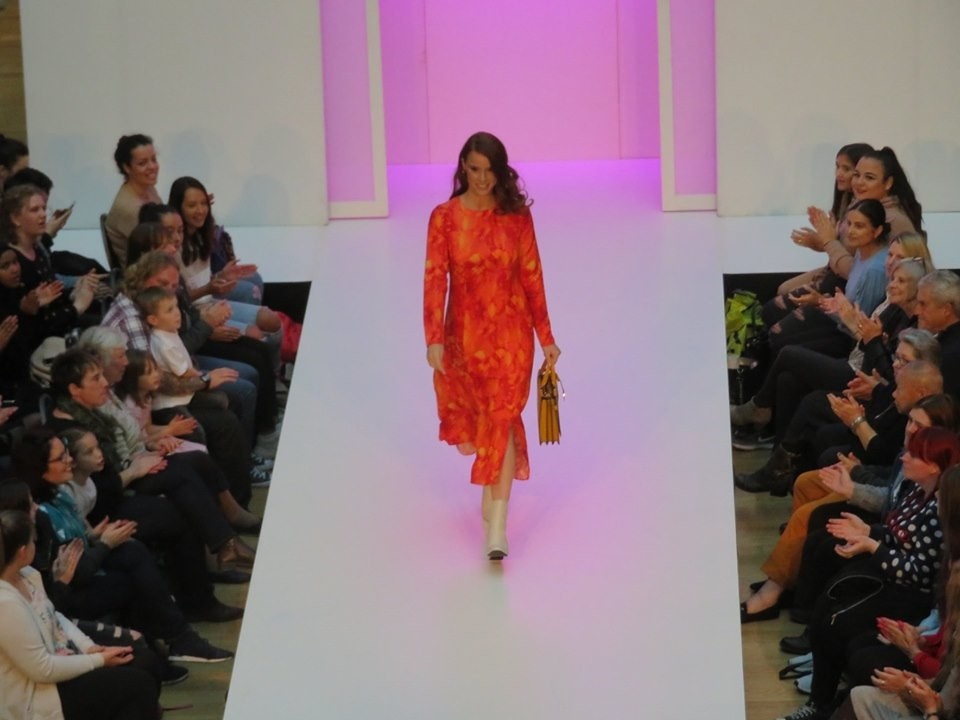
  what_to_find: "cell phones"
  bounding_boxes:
[60,200,76,217]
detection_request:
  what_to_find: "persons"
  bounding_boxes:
[0,129,289,719]
[733,144,960,720]
[420,130,563,558]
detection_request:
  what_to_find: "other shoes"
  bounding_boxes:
[727,322,846,720]
[154,367,284,720]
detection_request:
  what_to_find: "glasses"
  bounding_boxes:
[46,447,70,463]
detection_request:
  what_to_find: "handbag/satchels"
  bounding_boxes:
[537,356,566,447]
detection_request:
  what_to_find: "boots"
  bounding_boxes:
[481,485,494,522]
[485,499,509,560]
